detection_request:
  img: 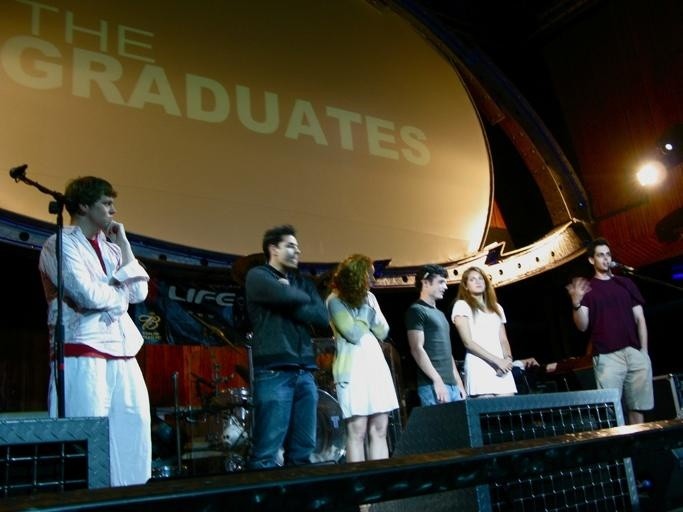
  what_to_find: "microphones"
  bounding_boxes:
[9,162,29,180]
[610,259,640,280]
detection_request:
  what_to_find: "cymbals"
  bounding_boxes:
[188,312,240,355]
[235,363,250,383]
[191,372,215,388]
[231,253,268,286]
[314,271,336,303]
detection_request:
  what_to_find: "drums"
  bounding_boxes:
[274,389,347,464]
[164,410,223,459]
[314,351,337,398]
[207,388,251,453]
[150,425,187,478]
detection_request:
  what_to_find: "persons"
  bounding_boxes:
[39,175,153,489]
[565,237,654,424]
[406,263,468,406]
[243,225,330,471]
[450,267,519,398]
[325,253,399,463]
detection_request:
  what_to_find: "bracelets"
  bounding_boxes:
[574,304,581,311]
